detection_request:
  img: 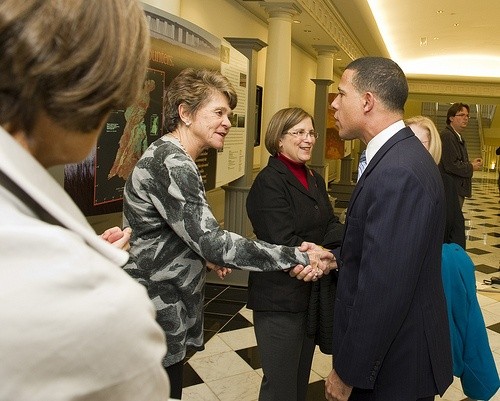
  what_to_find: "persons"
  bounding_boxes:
[122,65,337,401]
[283,56,454,401]
[245,107,343,401]
[0,1,171,401]
[403,115,467,252]
[440,102,483,209]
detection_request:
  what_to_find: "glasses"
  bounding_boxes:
[283,129,320,139]
[452,114,469,117]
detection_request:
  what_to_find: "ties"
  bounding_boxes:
[357,150,367,183]
